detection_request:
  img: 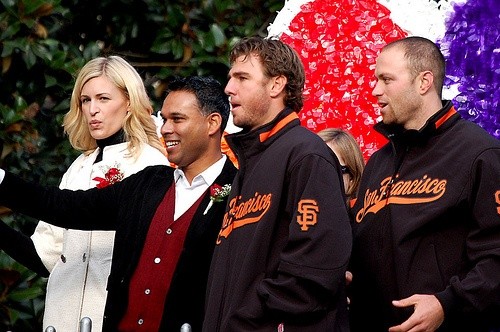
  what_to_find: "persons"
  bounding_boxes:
[315,129,366,332]
[200,39,352,332]
[1,74,243,332]
[1,55,171,332]
[348,35,500,332]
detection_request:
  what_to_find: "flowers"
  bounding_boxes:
[92,161,125,189]
[203,184,232,215]
